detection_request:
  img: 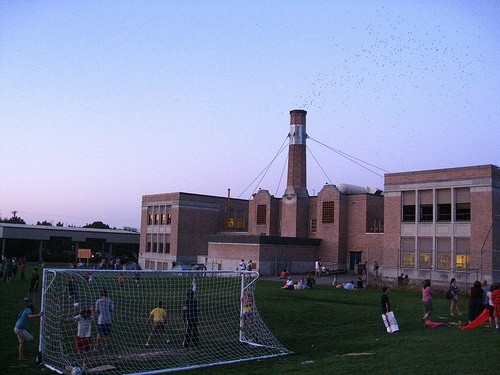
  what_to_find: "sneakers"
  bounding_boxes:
[103,346,111,351]
[166,340,171,344]
[145,343,149,346]
[93,346,101,351]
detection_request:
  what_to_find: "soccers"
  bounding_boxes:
[74,303,79,307]
[71,367,82,375]
[25,297,28,301]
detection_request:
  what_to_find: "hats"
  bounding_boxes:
[424,279,430,286]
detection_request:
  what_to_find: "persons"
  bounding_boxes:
[361,261,368,281]
[118,273,125,292]
[381,287,390,331]
[64,276,80,307]
[373,260,379,279]
[332,275,363,290]
[280,266,288,279]
[144,301,170,347]
[98,256,121,270]
[315,258,322,276]
[28,268,40,303]
[70,261,84,268]
[0,254,26,283]
[181,290,202,348]
[83,271,98,288]
[61,304,97,366]
[421,279,433,322]
[193,267,199,296]
[239,259,246,278]
[449,278,462,316]
[398,273,410,290]
[132,262,142,287]
[468,280,500,328]
[14,303,44,362]
[246,260,252,279]
[280,274,316,290]
[93,289,115,351]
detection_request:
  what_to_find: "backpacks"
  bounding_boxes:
[446,285,454,299]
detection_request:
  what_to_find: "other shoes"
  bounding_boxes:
[421,318,426,322]
[484,324,494,329]
[495,325,500,329]
